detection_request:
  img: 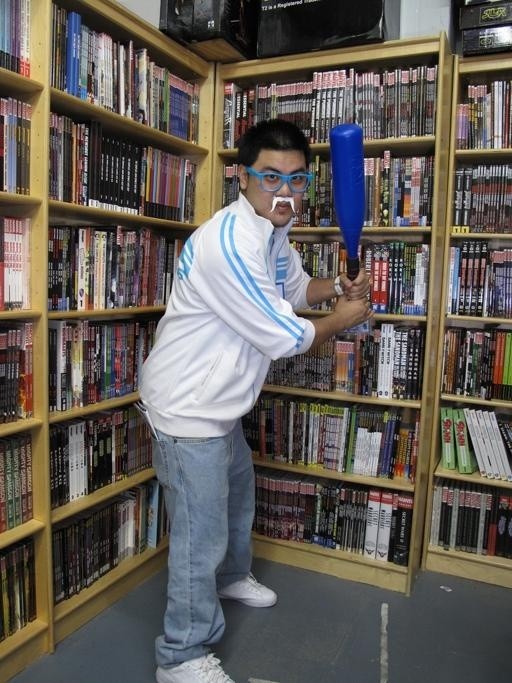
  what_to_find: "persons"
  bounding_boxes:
[136,118,370,682]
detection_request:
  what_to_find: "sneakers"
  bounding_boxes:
[215,569,279,609]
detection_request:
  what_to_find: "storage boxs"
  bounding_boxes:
[460,0,512,56]
[158,0,249,64]
[249,0,400,60]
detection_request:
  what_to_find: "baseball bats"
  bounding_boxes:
[329,122,367,282]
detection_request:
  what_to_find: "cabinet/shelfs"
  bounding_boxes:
[210,31,454,598]
[0,0,217,683]
[420,52,512,590]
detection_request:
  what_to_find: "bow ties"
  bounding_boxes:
[154,653,236,683]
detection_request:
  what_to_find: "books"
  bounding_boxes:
[219,62,437,565]
[0,0,202,646]
[428,81,511,559]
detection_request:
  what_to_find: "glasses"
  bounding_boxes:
[244,166,313,194]
